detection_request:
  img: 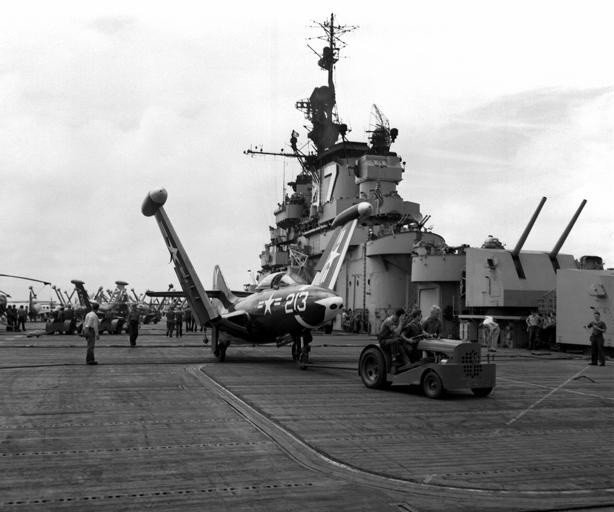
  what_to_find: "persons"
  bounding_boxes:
[6,303,207,364]
[341,305,607,366]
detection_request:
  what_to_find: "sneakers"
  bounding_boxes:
[87,362,97,365]
[588,363,605,366]
